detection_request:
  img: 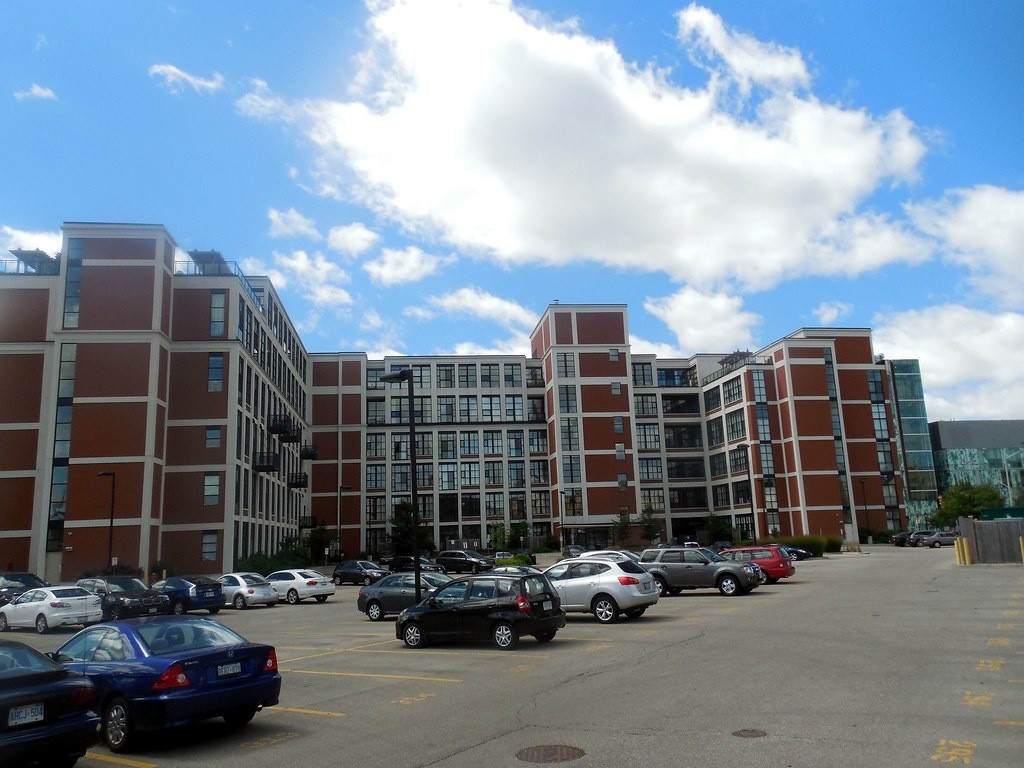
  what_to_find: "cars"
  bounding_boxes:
[333,560,393,587]
[264,568,337,605]
[717,544,795,586]
[541,557,663,624]
[390,556,446,577]
[149,576,229,617]
[889,531,959,548]
[214,571,281,611]
[41,612,282,758]
[563,545,641,562]
[0,638,104,768]
[394,573,568,651]
[714,541,733,553]
[682,542,699,548]
[489,565,554,574]
[766,542,813,561]
[0,584,104,635]
[74,574,172,622]
[495,551,514,566]
[356,570,490,622]
[0,570,51,612]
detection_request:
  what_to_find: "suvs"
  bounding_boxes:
[636,545,766,597]
[435,550,494,575]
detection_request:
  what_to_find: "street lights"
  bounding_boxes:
[859,478,871,534]
[382,369,424,606]
[756,477,768,537]
[558,490,567,548]
[97,469,116,572]
[338,485,351,560]
